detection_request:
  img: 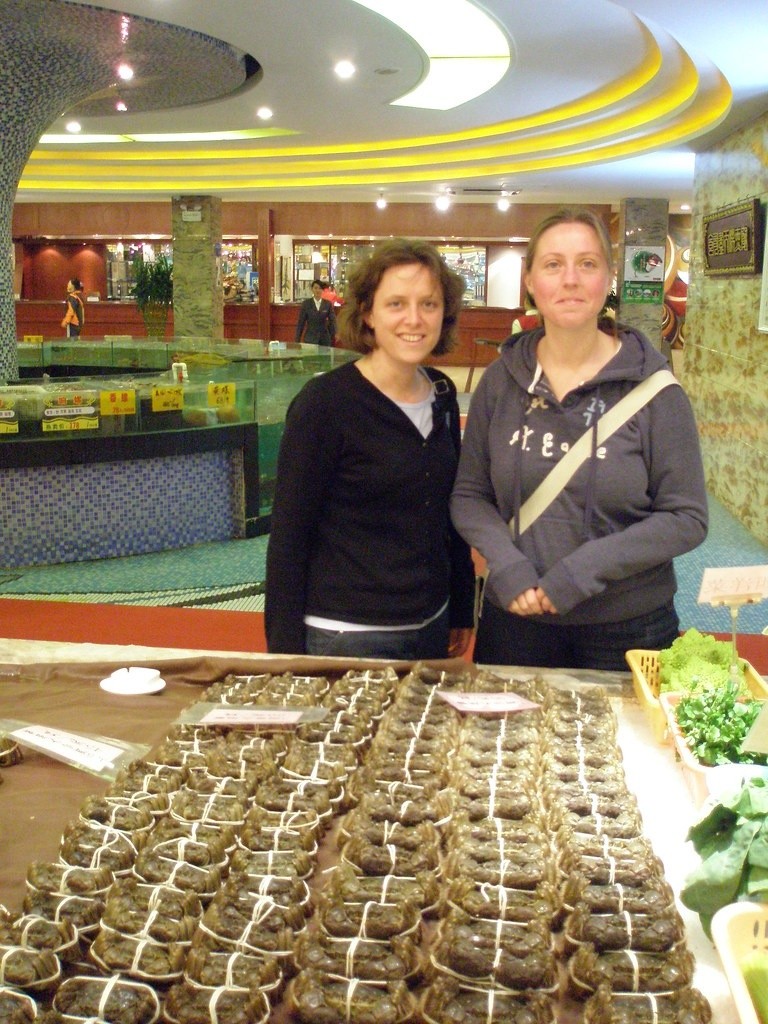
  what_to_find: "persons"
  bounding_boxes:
[511,292,540,334]
[295,280,345,347]
[451,209,710,672]
[61,279,84,337]
[264,237,474,658]
